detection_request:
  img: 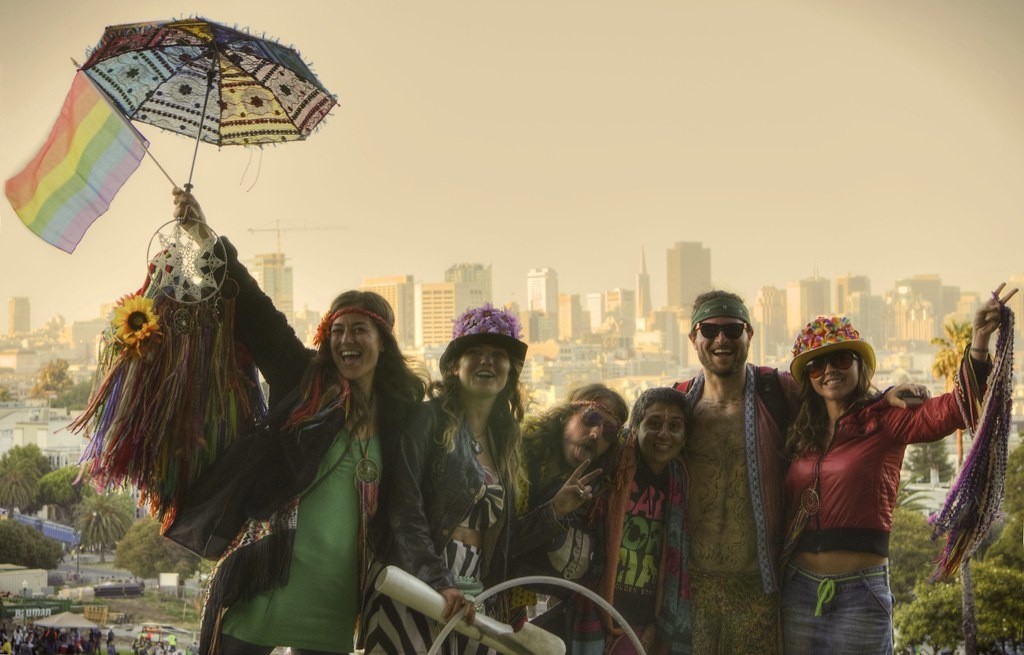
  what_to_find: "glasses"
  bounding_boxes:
[579,407,620,444]
[693,322,747,340]
[804,350,862,378]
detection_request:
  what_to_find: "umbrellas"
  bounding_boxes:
[76,16,340,225]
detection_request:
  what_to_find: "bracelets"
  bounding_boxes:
[970,347,989,352]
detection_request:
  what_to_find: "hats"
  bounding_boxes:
[789,314,876,389]
[447,304,528,378]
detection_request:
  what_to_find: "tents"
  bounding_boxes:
[33,611,97,629]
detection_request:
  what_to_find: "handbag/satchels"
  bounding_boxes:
[161,381,303,560]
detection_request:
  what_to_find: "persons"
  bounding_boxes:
[781,282,1019,655]
[68,570,136,585]
[657,291,932,655]
[130,626,199,655]
[365,308,530,655]
[607,387,689,655]
[170,182,426,655]
[0,620,119,655]
[524,383,630,655]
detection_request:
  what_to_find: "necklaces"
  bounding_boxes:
[471,429,486,453]
[353,418,379,484]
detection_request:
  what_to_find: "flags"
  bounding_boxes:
[4,73,151,254]
